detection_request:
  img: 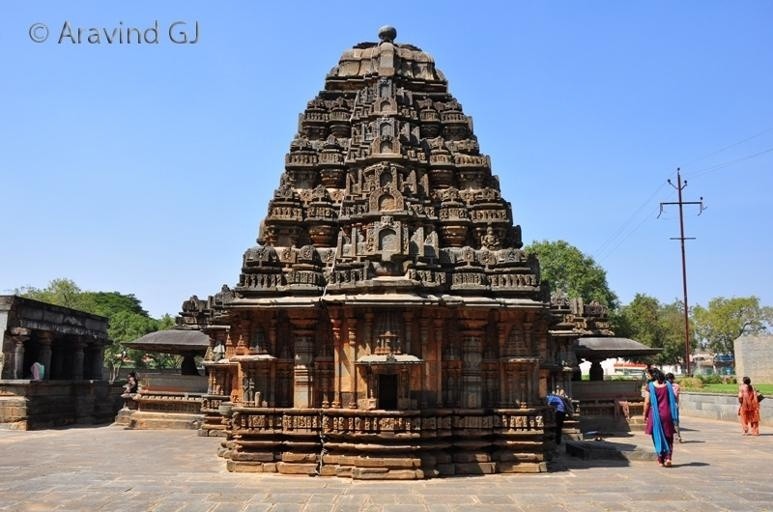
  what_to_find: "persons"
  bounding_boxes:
[737,376,759,434]
[664,371,683,444]
[644,368,678,465]
[547,394,564,444]
[481,226,501,252]
[128,372,138,393]
